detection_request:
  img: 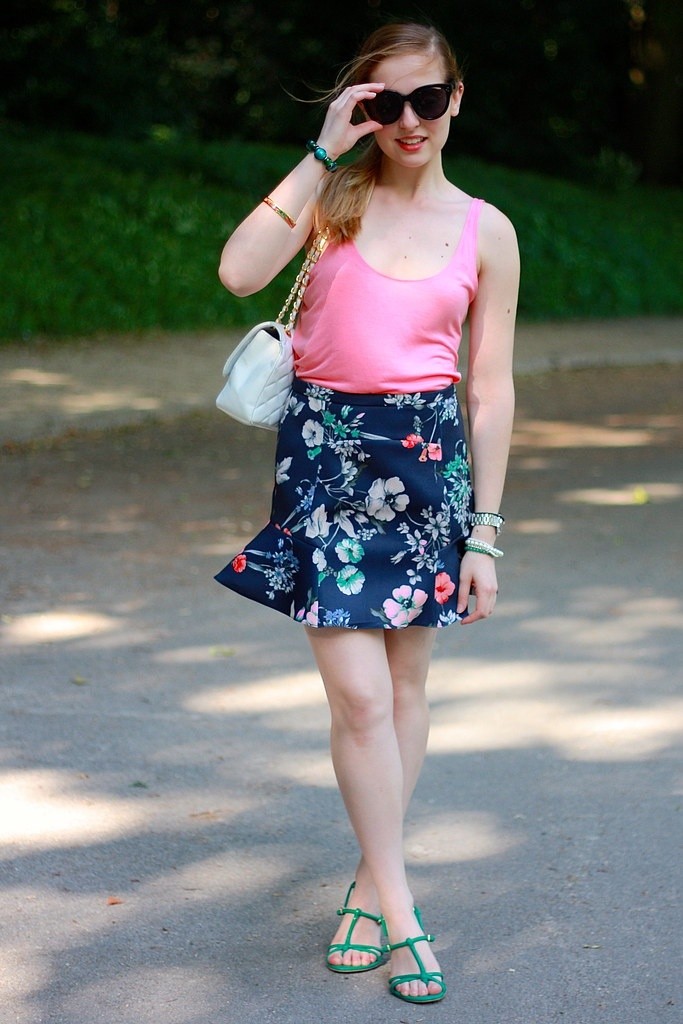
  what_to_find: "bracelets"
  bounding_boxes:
[306,140,338,173]
[263,196,297,229]
[463,537,504,559]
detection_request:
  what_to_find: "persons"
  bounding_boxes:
[210,20,519,1005]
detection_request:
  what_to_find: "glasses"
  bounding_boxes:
[360,83,453,125]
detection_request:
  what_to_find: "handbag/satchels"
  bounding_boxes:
[215,321,296,432]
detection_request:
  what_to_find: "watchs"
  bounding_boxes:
[469,511,504,535]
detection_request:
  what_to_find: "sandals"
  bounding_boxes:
[325,882,383,972]
[380,903,446,1002]
